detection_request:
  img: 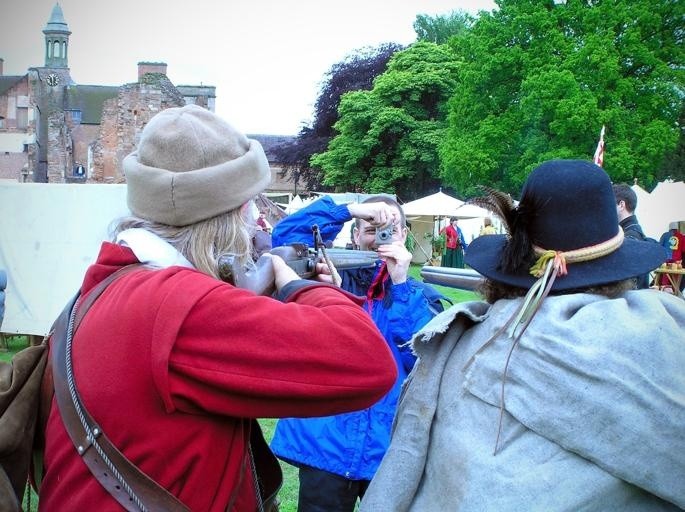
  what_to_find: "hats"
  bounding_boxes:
[118,101,274,231]
[463,154,675,293]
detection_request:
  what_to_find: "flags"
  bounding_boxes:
[592,140,604,168]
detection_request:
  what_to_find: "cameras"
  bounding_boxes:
[376,221,393,245]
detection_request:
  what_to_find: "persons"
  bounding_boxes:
[439,216,468,269]
[36,103,401,512]
[658,222,685,295]
[266,193,445,512]
[479,218,500,236]
[613,183,650,290]
[360,159,684,512]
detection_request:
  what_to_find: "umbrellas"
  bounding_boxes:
[400,187,489,236]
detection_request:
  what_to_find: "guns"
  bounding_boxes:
[236,243,379,300]
[420,265,487,292]
